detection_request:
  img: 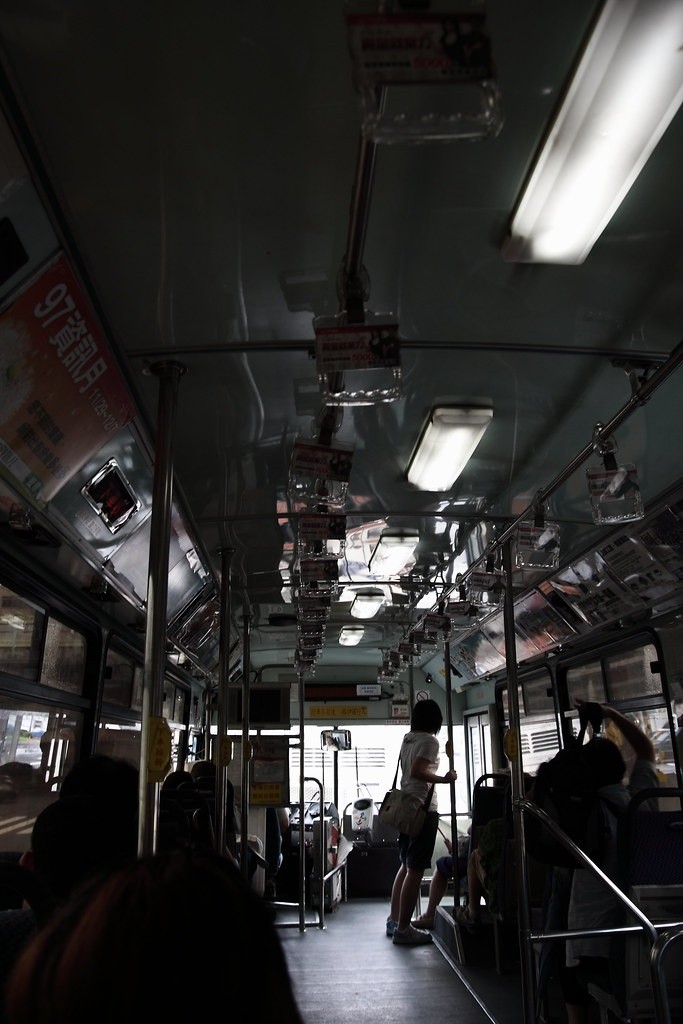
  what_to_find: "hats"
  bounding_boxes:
[236,834,270,869]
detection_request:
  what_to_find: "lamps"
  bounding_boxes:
[495,0,683,265]
[337,624,364,647]
[404,402,495,491]
[349,592,385,619]
[368,534,420,576]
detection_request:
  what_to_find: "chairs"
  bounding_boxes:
[487,777,548,923]
[32,794,127,901]
[586,786,683,1024]
[265,808,284,877]
[194,776,235,834]
[468,772,510,854]
[160,790,213,848]
[158,799,188,850]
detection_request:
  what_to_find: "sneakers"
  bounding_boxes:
[453,905,481,934]
[411,915,434,931]
[386,917,398,935]
[392,922,432,944]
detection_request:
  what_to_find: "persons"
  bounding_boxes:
[386,697,660,1024]
[0,749,304,1024]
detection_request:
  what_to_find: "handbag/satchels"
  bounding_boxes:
[378,788,427,837]
